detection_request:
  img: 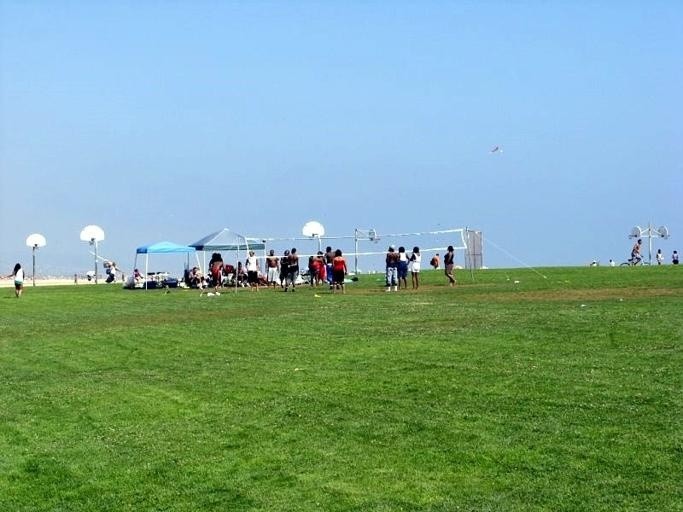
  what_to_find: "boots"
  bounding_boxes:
[392,286,398,291]
[385,287,390,292]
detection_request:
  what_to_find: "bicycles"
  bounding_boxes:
[619,255,649,266]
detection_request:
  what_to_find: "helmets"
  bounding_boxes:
[284,250,289,254]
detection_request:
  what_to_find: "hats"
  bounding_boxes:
[390,245,395,249]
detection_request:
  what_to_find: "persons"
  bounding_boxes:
[630,239,642,266]
[433,254,439,269]
[670,250,679,264]
[655,248,664,265]
[592,260,597,266]
[5,263,25,298]
[74,273,77,285]
[609,259,614,266]
[443,246,456,288]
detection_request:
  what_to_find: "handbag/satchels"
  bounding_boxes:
[430,258,434,265]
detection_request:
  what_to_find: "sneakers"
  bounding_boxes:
[215,291,221,296]
[448,280,456,287]
[206,292,214,297]
